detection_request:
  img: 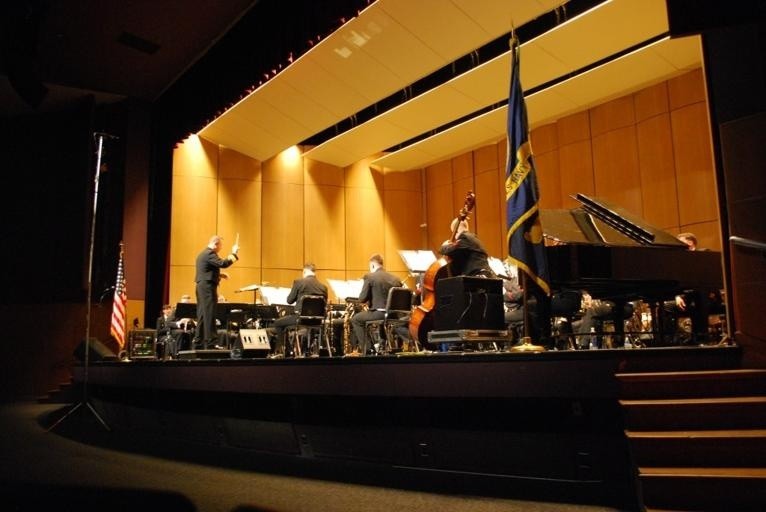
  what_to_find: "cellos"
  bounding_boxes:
[408,190,476,350]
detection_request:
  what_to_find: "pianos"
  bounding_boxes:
[539,191,722,346]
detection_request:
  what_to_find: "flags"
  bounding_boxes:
[109,250,128,351]
[506,38,554,345]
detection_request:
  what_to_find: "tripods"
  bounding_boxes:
[43,134,114,433]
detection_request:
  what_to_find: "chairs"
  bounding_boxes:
[283,295,333,358]
[363,287,414,356]
[510,296,726,346]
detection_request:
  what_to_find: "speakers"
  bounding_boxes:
[232,328,271,358]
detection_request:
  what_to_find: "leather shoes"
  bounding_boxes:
[207,344,224,350]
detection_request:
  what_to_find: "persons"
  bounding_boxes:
[352,255,402,354]
[657,232,722,346]
[193,235,241,348]
[275,263,328,356]
[167,295,194,357]
[577,299,636,349]
[503,288,524,346]
[218,295,227,303]
[156,305,175,360]
[549,289,582,317]
[437,217,487,277]
